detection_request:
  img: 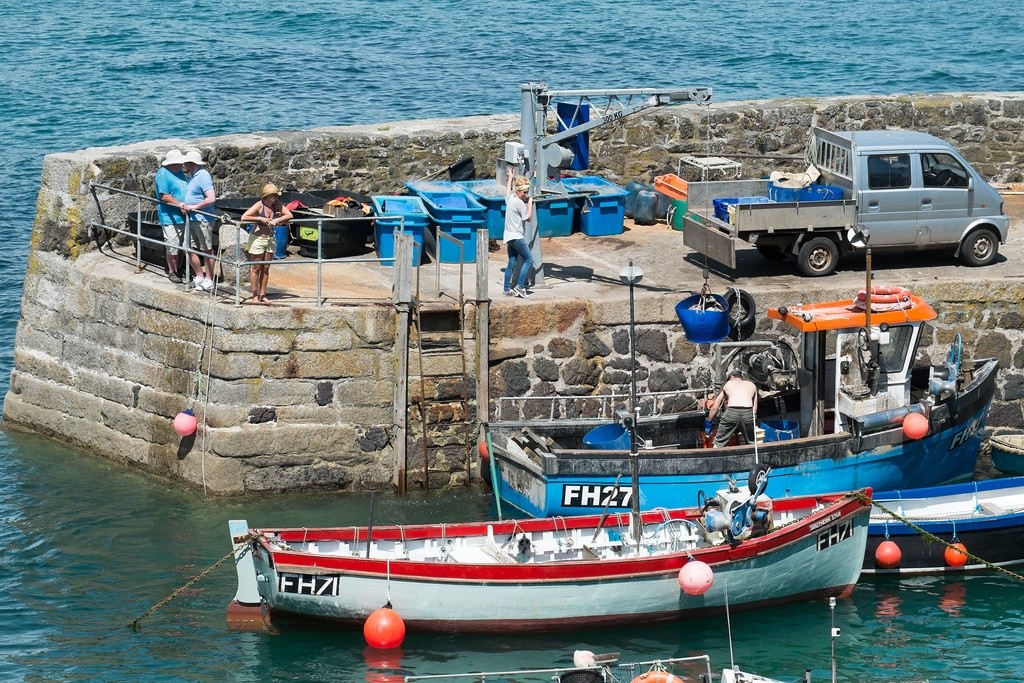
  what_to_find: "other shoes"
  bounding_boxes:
[169,272,182,283]
[252,295,259,302]
[260,296,270,303]
[165,265,183,278]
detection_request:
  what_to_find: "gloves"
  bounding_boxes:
[704,417,713,435]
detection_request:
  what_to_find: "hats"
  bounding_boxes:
[260,184,282,198]
[161,150,186,166]
[726,370,741,382]
[183,151,206,166]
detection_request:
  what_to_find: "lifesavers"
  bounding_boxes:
[857,286,911,303]
[853,296,911,312]
[723,288,756,327]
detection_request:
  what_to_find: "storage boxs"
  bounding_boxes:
[654,173,689,201]
[713,182,845,225]
[127,175,633,273]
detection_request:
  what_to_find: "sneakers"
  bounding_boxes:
[194,277,205,288]
[200,278,215,291]
[503,290,518,297]
[513,285,530,298]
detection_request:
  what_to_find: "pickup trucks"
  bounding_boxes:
[683,125,1009,277]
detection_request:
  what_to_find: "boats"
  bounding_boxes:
[404,596,841,683]
[855,476,1024,584]
[479,292,1001,519]
[224,262,874,628]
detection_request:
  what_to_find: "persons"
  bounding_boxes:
[705,371,758,449]
[241,184,293,306]
[155,150,191,283]
[503,168,534,299]
[180,151,215,291]
